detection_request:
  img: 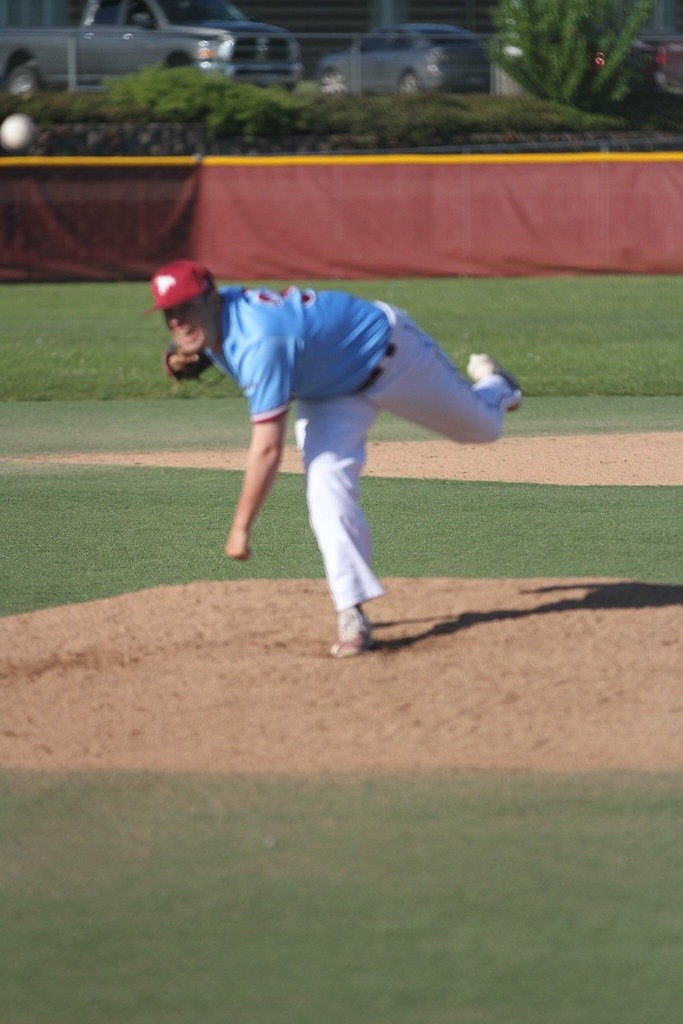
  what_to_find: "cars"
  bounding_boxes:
[317,25,494,94]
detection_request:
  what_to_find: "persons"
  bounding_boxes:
[150,260,525,661]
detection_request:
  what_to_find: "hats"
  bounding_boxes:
[143,260,215,317]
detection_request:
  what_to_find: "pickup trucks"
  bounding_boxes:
[1,0,303,98]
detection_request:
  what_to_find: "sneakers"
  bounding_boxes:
[330,605,371,658]
[466,353,521,412]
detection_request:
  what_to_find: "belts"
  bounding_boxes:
[347,343,397,396]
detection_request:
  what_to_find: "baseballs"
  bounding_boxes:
[0,110,39,153]
